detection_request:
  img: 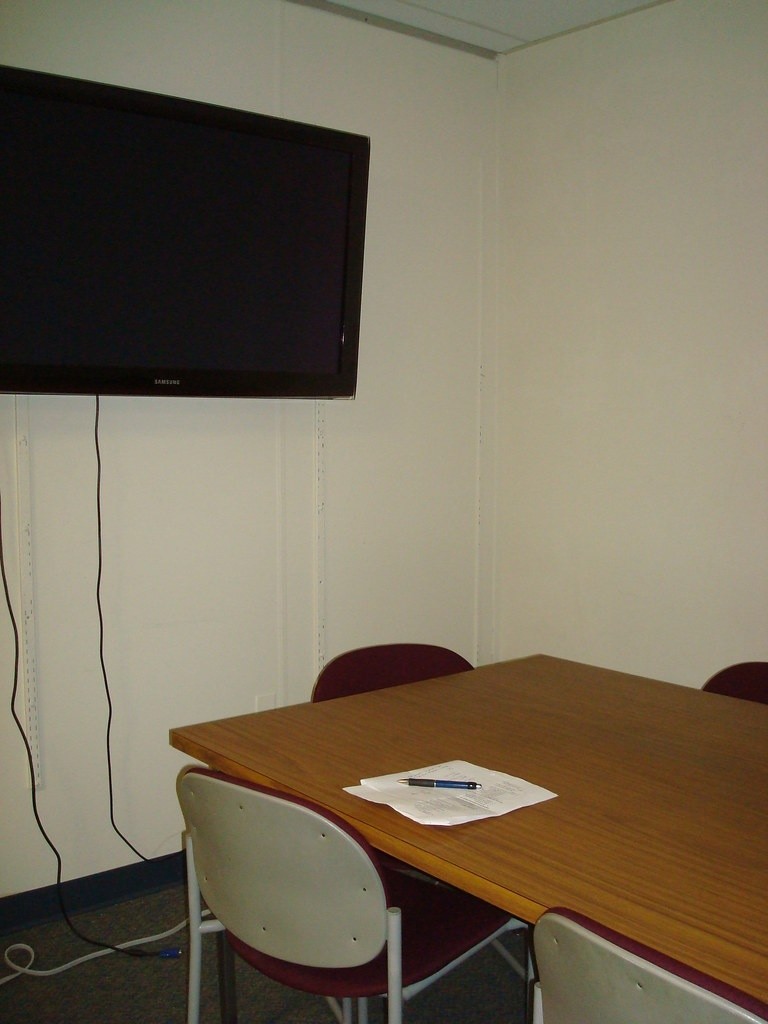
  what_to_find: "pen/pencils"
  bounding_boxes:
[397,778,481,790]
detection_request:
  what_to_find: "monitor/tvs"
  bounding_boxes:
[0,66,372,398]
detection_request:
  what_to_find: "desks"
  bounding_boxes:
[170,653,768,1024]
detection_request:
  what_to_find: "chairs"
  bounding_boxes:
[700,661,768,704]
[177,764,537,1024]
[530,907,768,1024]
[309,643,474,702]
